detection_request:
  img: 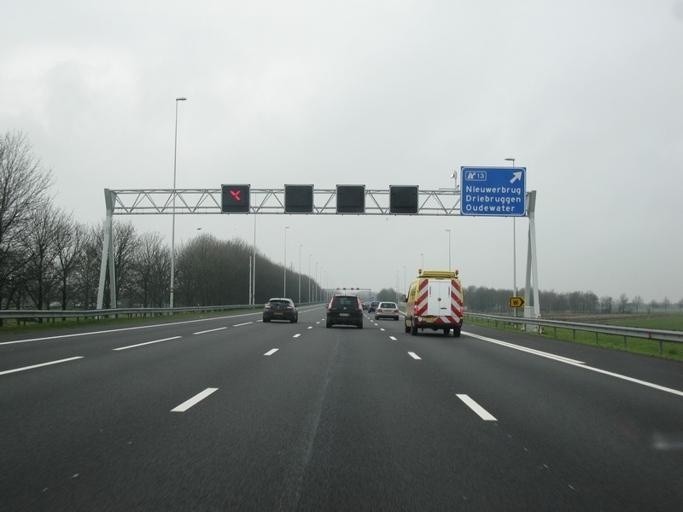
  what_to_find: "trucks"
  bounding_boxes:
[401,267,465,338]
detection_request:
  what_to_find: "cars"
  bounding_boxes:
[360,300,401,323]
[49,300,101,310]
[260,294,300,324]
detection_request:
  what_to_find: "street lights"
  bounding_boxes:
[283,223,332,306]
[446,226,453,273]
[168,95,189,312]
[502,156,519,327]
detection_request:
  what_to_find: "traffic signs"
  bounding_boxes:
[460,164,526,217]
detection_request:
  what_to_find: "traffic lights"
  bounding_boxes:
[283,184,313,214]
[220,182,251,214]
[389,185,418,215]
[336,183,367,214]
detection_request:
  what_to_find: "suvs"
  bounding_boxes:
[325,296,365,330]
